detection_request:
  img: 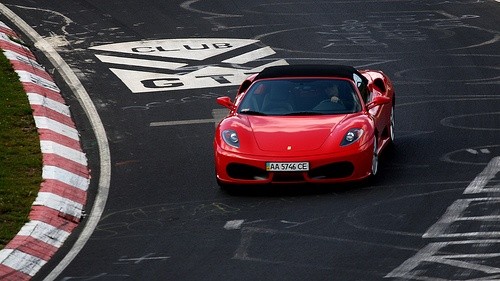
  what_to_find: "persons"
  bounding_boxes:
[302,82,353,111]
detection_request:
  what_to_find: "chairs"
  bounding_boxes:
[262,81,290,115]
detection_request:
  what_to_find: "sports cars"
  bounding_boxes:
[213,64,396,193]
